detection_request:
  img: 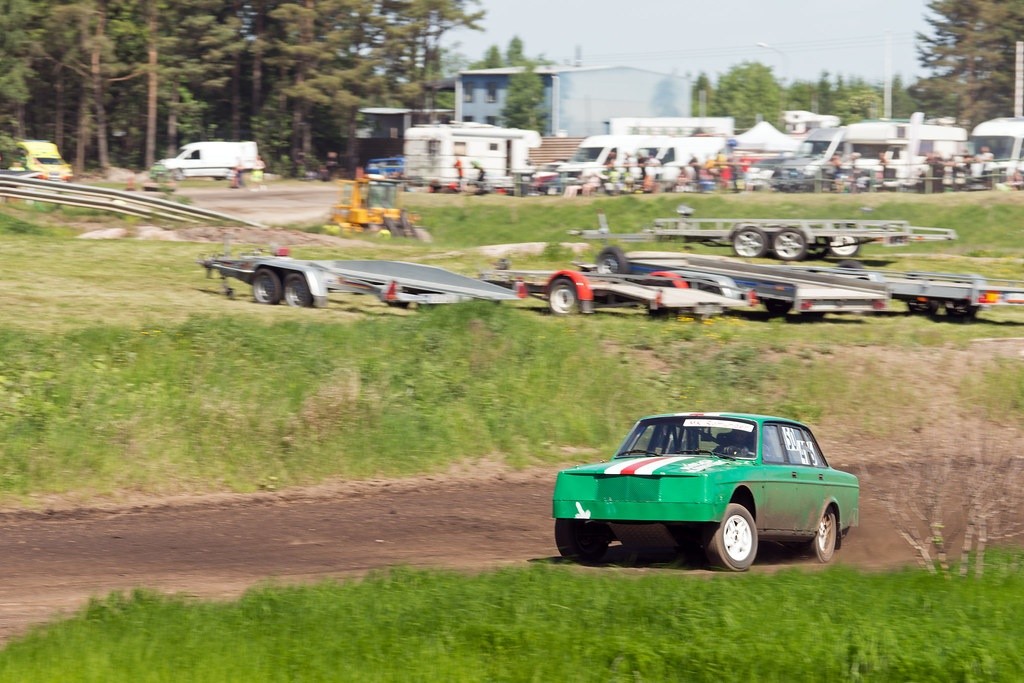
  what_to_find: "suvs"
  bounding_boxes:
[364,157,406,183]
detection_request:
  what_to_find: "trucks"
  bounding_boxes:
[402,120,545,197]
[156,140,261,182]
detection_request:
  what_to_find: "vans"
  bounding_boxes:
[8,140,74,186]
[779,126,911,191]
[555,133,681,193]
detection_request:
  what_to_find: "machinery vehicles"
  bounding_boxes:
[320,171,436,249]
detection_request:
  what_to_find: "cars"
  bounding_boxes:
[550,410,862,569]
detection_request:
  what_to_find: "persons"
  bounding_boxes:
[226,144,1024,194]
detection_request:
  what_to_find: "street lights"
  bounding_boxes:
[754,42,793,88]
